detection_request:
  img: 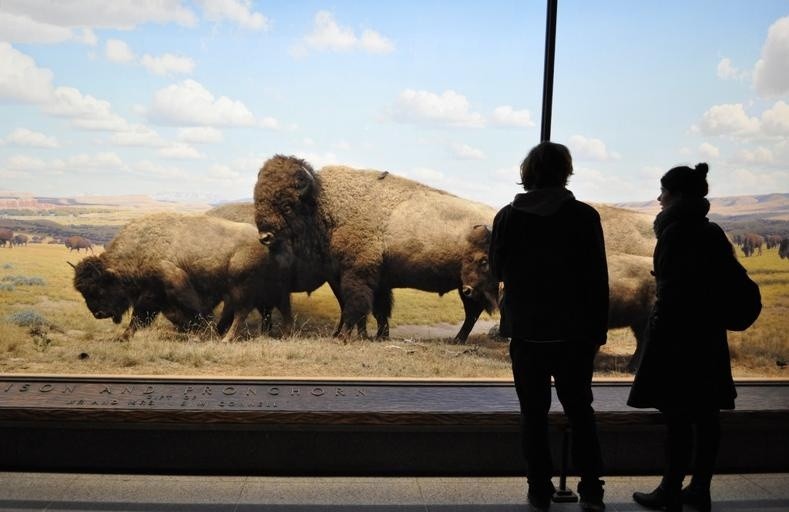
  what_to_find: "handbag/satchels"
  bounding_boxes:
[702,242,761,331]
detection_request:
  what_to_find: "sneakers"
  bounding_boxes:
[632,486,711,510]
[579,490,605,512]
[528,489,551,511]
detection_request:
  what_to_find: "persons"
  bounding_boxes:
[628,163,739,511]
[488,144,610,512]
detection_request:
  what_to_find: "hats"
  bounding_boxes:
[661,163,708,198]
